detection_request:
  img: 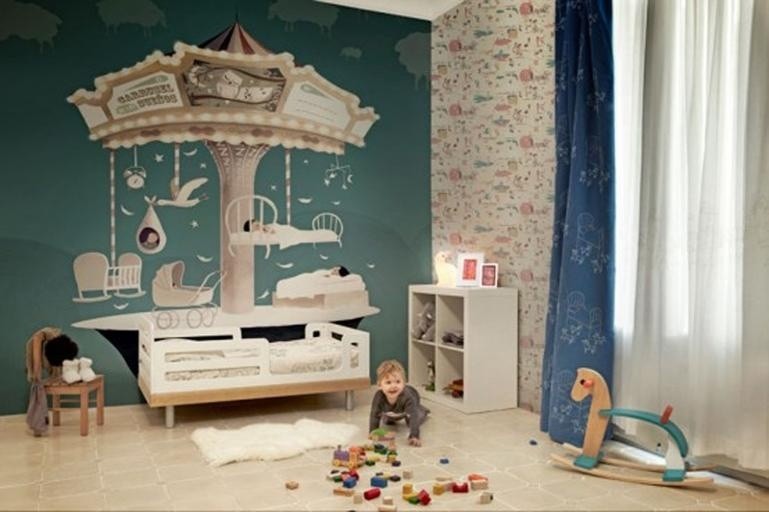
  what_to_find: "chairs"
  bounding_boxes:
[30,326,106,438]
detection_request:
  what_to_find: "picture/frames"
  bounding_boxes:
[478,262,498,288]
[455,251,485,287]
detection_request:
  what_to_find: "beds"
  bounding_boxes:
[224,194,346,260]
[136,313,373,429]
[271,270,369,313]
[71,252,147,305]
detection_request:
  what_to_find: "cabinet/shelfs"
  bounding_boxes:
[407,283,519,414]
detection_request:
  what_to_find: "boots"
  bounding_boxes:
[63,357,97,383]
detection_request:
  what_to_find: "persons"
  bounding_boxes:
[370,360,430,447]
[243,219,299,233]
[276,265,350,291]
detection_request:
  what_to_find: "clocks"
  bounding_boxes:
[121,165,148,193]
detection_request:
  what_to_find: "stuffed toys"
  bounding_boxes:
[412,302,435,341]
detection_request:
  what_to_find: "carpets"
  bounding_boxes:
[189,417,361,471]
